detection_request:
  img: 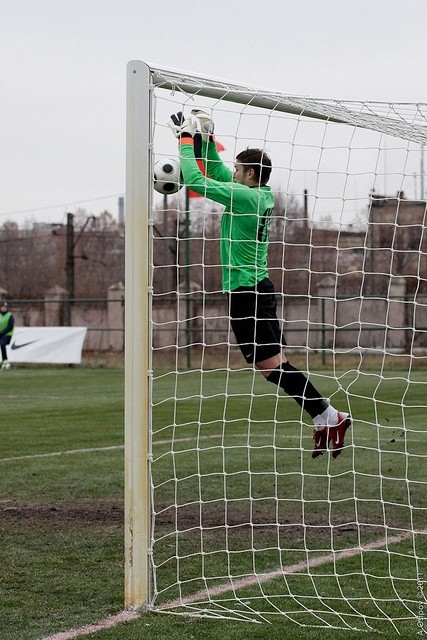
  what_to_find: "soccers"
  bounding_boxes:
[152,158,183,194]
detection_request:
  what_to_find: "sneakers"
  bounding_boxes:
[330,412,352,458]
[312,424,331,458]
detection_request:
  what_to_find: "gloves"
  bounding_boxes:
[191,109,215,134]
[167,111,197,138]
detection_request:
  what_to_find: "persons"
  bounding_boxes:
[0,302,15,370]
[170,111,352,460]
